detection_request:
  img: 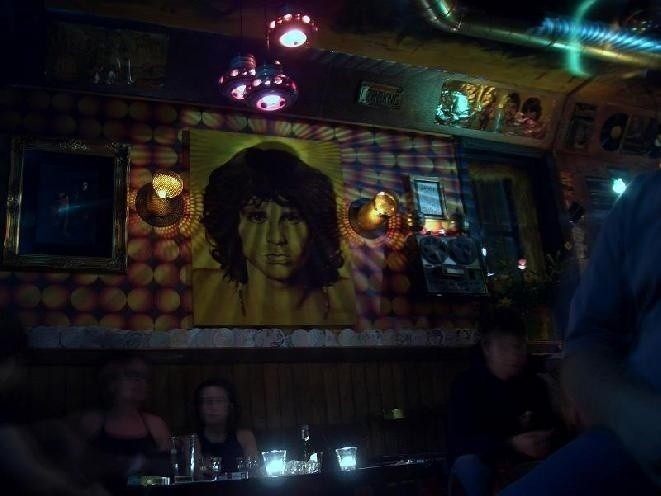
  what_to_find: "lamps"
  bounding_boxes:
[343,189,401,234]
[214,0,322,116]
[144,164,188,217]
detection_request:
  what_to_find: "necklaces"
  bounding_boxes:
[234,279,330,320]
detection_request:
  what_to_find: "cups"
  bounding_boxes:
[209,456,257,480]
[335,445,360,471]
[261,448,288,480]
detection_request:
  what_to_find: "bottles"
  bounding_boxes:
[300,424,314,463]
[167,434,202,480]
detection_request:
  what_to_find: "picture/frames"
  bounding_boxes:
[1,131,135,278]
[407,172,450,220]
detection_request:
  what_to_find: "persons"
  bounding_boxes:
[180,380,259,462]
[497,165,661,496]
[474,86,541,138]
[70,349,172,456]
[200,140,352,322]
[0,307,106,495]
[441,323,568,496]
